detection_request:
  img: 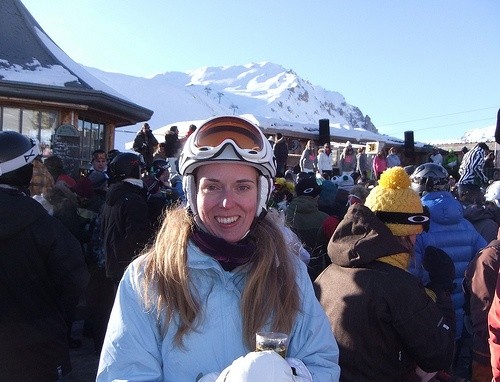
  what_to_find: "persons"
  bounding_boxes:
[97,116,340,382]
[0,124,198,382]
[266,109,500,382]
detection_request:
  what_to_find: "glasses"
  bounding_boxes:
[189,116,264,160]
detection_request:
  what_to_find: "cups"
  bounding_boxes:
[255,331,289,359]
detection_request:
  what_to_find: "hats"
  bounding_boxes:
[338,175,354,192]
[363,163,424,236]
[320,180,338,199]
[298,179,321,197]
[1,129,39,172]
[330,176,338,184]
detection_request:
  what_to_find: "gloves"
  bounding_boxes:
[216,351,311,382]
[422,245,457,292]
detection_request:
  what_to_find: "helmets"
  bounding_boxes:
[178,117,278,175]
[409,162,450,196]
